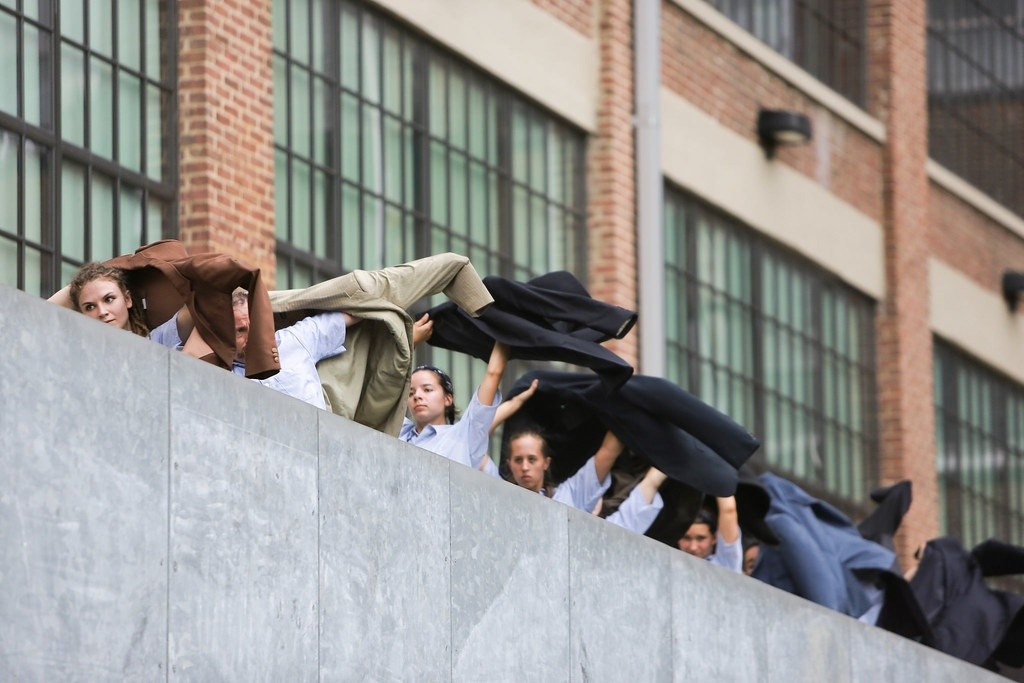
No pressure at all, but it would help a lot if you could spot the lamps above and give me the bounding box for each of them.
[758,109,814,144]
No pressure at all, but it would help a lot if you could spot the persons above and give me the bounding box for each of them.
[196,253,494,439]
[47,240,281,380]
[741,544,759,573]
[489,368,760,512]
[677,487,745,572]
[599,466,668,536]
[399,272,639,470]
